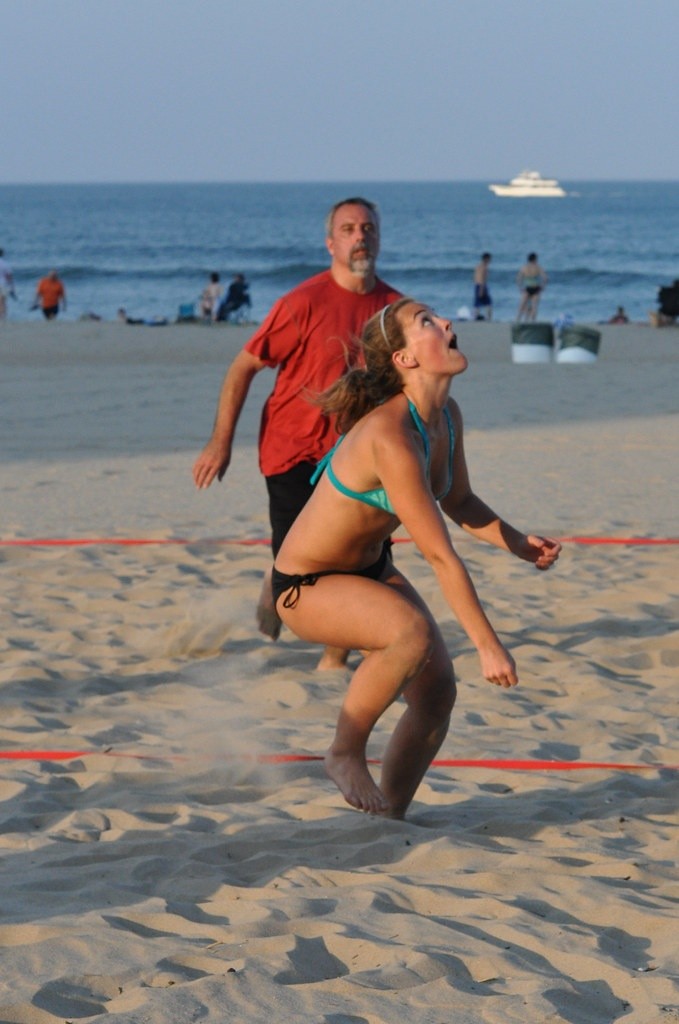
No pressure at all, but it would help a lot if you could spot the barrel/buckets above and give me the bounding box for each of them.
[555,323,602,365]
[511,323,555,363]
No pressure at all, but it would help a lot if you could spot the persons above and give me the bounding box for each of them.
[34,270,67,320]
[216,273,252,322]
[0,249,14,314]
[656,279,679,322]
[118,309,127,319]
[517,254,545,322]
[199,273,223,320]
[195,196,409,670]
[474,253,492,318]
[271,298,563,817]
[608,307,628,323]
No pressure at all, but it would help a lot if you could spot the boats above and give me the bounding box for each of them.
[489,169,566,197]
